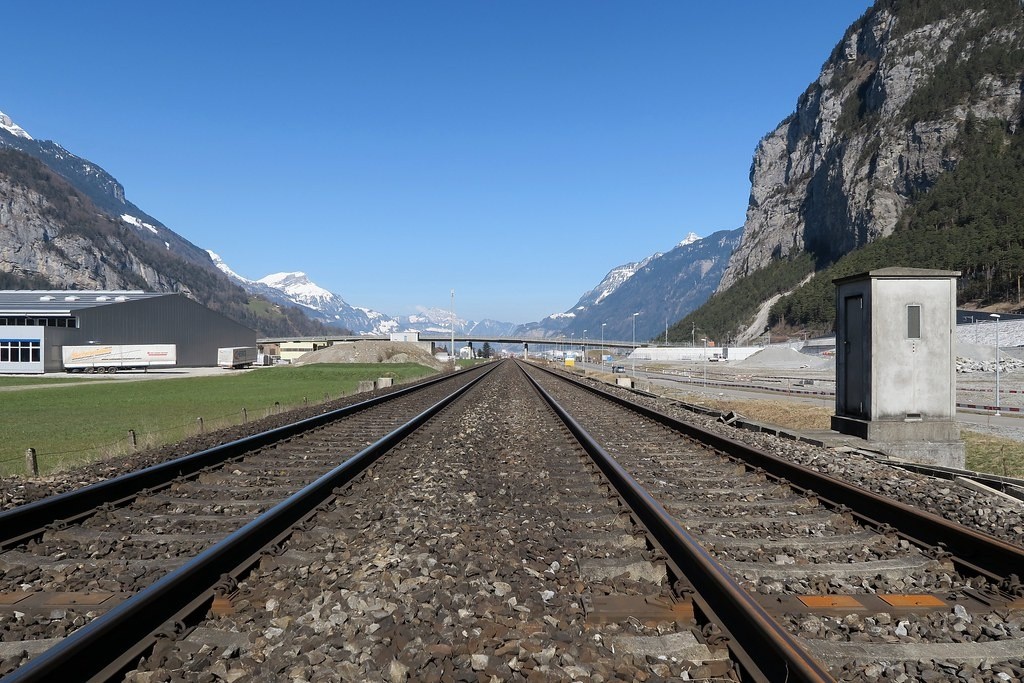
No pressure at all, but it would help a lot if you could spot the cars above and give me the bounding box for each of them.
[616,366,625,373]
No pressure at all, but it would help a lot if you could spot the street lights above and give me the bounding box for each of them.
[601,323,606,373]
[692,321,695,347]
[450,288,456,357]
[555,330,588,365]
[989,314,1001,416]
[632,313,640,376]
[701,339,706,386]
